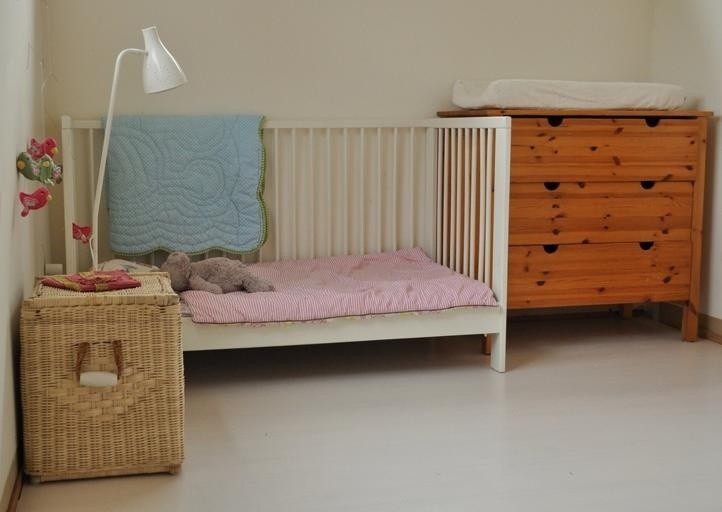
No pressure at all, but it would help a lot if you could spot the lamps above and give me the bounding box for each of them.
[85,22,189,275]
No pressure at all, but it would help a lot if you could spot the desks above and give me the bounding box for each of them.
[19,271,185,485]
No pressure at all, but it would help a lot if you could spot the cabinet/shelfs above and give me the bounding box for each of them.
[436,107,713,354]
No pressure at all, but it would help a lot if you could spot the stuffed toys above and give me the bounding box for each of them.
[17,137,63,217]
[149,252,275,295]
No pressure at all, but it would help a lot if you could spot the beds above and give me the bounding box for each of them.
[61,113,511,376]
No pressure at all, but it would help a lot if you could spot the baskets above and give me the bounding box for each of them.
[20,306,187,486]
